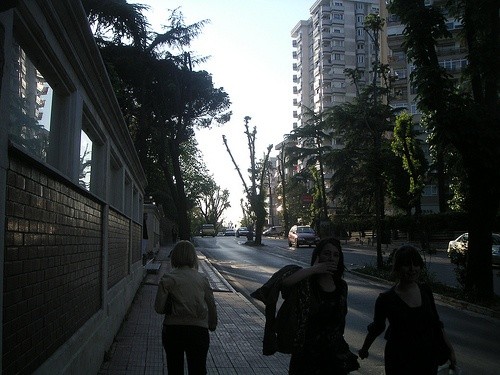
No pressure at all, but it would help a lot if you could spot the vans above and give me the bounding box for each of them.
[262,225,285,237]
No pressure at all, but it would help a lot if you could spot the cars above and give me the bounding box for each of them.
[217,231,225,237]
[236,227,256,238]
[288,224,321,248]
[446,232,500,265]
[224,228,236,236]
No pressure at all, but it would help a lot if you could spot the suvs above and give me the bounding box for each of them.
[200,224,216,238]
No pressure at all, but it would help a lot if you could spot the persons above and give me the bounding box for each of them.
[359,246,457,375]
[281,239,348,375]
[142,212,149,254]
[155,241,218,375]
